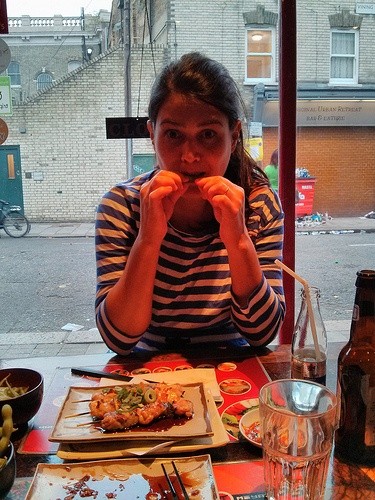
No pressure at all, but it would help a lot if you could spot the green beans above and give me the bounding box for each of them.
[0,403,18,471]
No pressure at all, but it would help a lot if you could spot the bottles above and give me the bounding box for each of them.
[333,270,375,467]
[290,286,328,388]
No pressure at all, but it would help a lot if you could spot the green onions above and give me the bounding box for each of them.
[102,385,156,413]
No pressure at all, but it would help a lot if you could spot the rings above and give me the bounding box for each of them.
[225,187,229,193]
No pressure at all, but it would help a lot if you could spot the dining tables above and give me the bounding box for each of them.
[0,342,375,500]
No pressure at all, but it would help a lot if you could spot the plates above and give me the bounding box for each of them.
[223,398,259,440]
[55,387,231,460]
[45,380,214,441]
[24,454,220,500]
[219,378,252,395]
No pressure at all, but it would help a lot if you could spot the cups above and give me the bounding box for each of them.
[257,379,337,500]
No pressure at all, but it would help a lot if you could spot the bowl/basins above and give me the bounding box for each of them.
[239,408,262,449]
[0,440,17,499]
[0,367,44,427]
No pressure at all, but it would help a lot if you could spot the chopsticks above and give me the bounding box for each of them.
[160,461,190,500]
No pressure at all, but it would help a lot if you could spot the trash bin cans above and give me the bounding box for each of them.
[294,175,316,216]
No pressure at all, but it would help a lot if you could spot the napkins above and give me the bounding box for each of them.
[100,368,222,403]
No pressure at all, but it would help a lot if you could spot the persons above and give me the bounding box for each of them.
[93,52,287,356]
[264,148,279,192]
